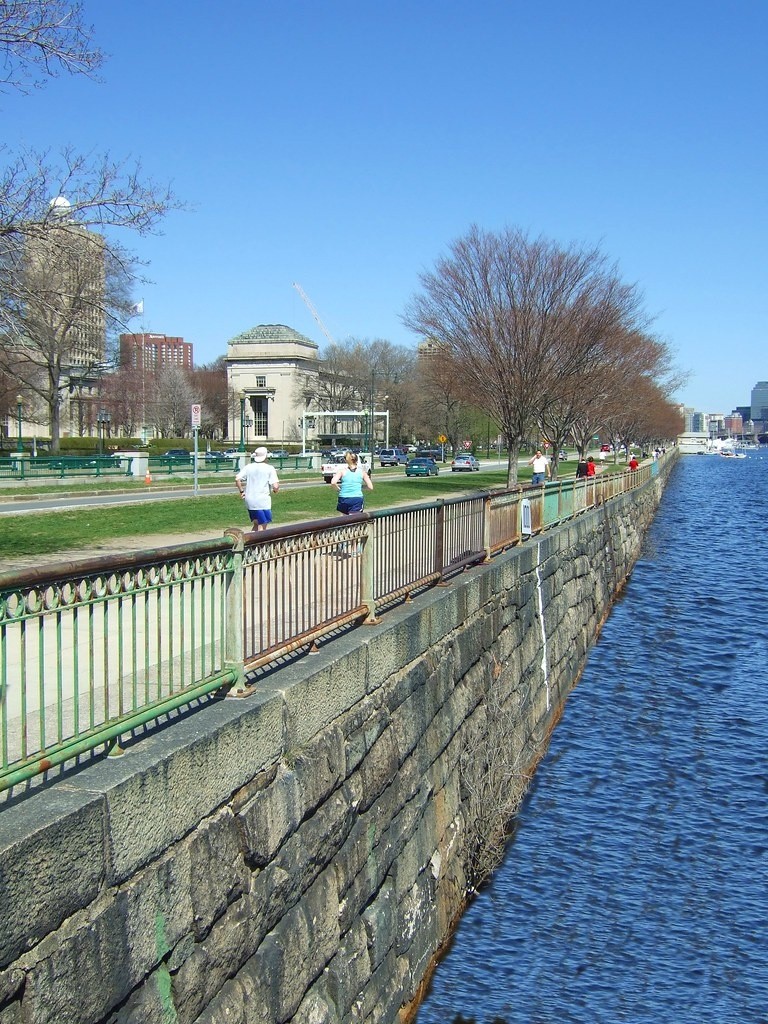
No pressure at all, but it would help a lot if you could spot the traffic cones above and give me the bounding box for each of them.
[144,469,151,484]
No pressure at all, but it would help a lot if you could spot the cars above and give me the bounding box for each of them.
[404,444,417,453]
[404,458,440,477]
[378,449,409,466]
[396,444,409,454]
[600,444,610,453]
[224,448,239,462]
[550,450,567,462]
[451,456,480,471]
[160,450,194,465]
[46,454,119,470]
[251,450,273,458]
[272,450,289,457]
[205,452,224,464]
[319,445,392,459]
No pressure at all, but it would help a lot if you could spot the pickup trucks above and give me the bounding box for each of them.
[415,445,448,463]
[299,449,322,457]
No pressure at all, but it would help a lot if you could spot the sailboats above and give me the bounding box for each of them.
[697,428,747,459]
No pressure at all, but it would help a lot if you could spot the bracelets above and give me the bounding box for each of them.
[240,490,244,493]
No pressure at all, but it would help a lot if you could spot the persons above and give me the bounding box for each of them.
[331,452,374,556]
[587,456,596,476]
[599,449,605,467]
[235,447,279,530]
[655,447,665,457]
[629,457,638,470]
[576,459,587,478]
[529,450,550,484]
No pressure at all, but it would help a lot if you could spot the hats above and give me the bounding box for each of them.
[254,446,267,463]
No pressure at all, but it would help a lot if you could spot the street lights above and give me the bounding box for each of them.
[370,371,398,470]
[16,395,24,453]
[237,391,246,452]
